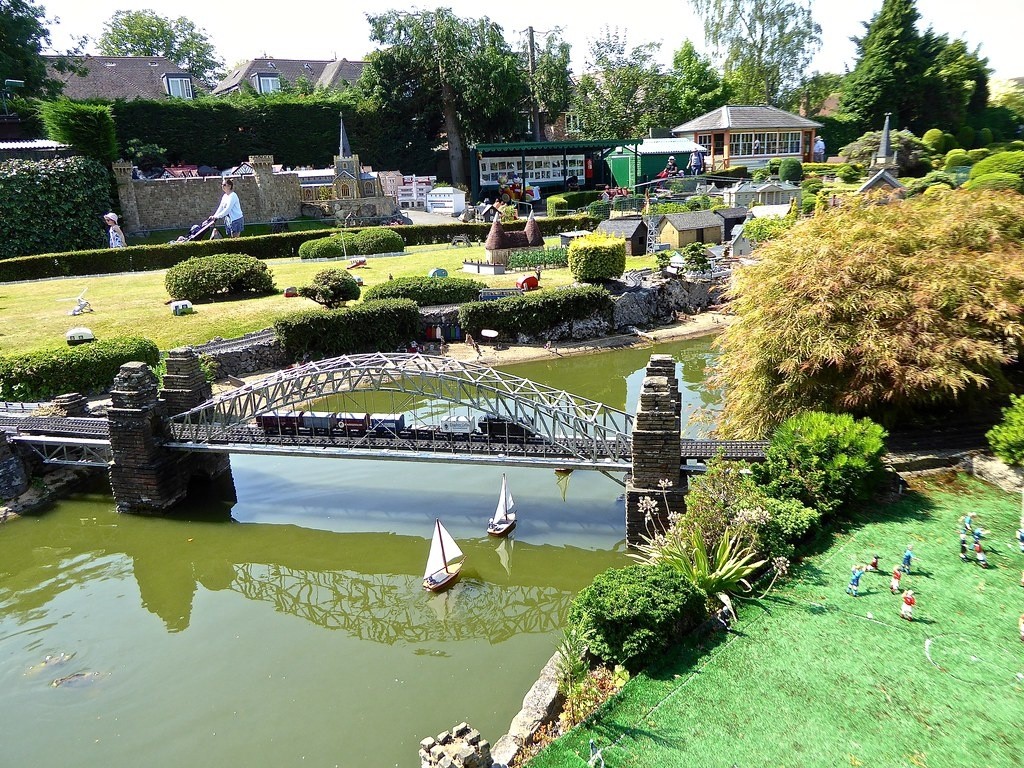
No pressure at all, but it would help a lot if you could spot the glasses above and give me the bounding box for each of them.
[104,218,110,222]
[220,183,226,187]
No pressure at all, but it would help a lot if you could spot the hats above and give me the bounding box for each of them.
[668,156,674,159]
[692,148,698,152]
[104,212,119,225]
[815,136,823,140]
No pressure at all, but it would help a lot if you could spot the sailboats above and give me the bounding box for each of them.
[485,471,518,537]
[421,517,467,592]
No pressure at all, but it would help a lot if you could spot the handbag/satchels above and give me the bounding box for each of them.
[210,228,224,240]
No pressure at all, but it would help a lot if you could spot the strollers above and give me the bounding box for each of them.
[170,217,219,243]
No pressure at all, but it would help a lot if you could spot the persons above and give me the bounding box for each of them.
[104,213,127,248]
[845,544,928,621]
[954,511,990,568]
[655,147,704,178]
[1015,517,1024,554]
[814,136,825,163]
[176,179,244,242]
[566,176,580,192]
